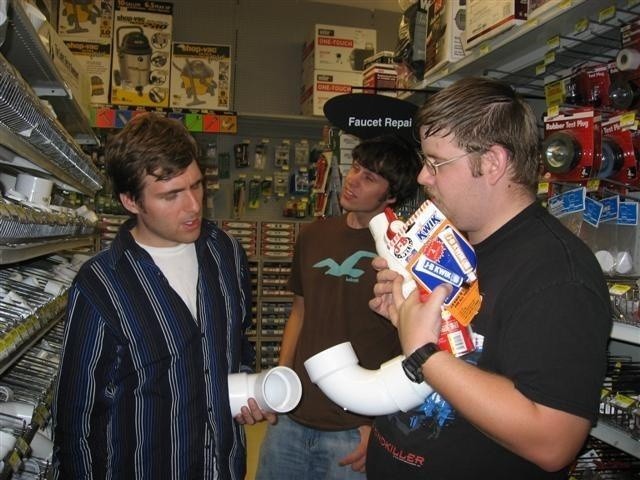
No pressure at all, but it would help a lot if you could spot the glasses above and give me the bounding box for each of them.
[416,148,483,176]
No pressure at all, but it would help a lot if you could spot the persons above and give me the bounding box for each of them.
[364,73,614,480]
[50,114,279,480]
[252,132,420,480]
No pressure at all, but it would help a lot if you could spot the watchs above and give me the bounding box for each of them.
[400,341,444,384]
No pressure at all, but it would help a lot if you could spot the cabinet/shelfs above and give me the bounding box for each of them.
[590,320,640,459]
[95,214,311,371]
[0,0,107,480]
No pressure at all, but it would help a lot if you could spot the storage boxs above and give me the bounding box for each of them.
[58,0,231,110]
[302,0,562,117]
